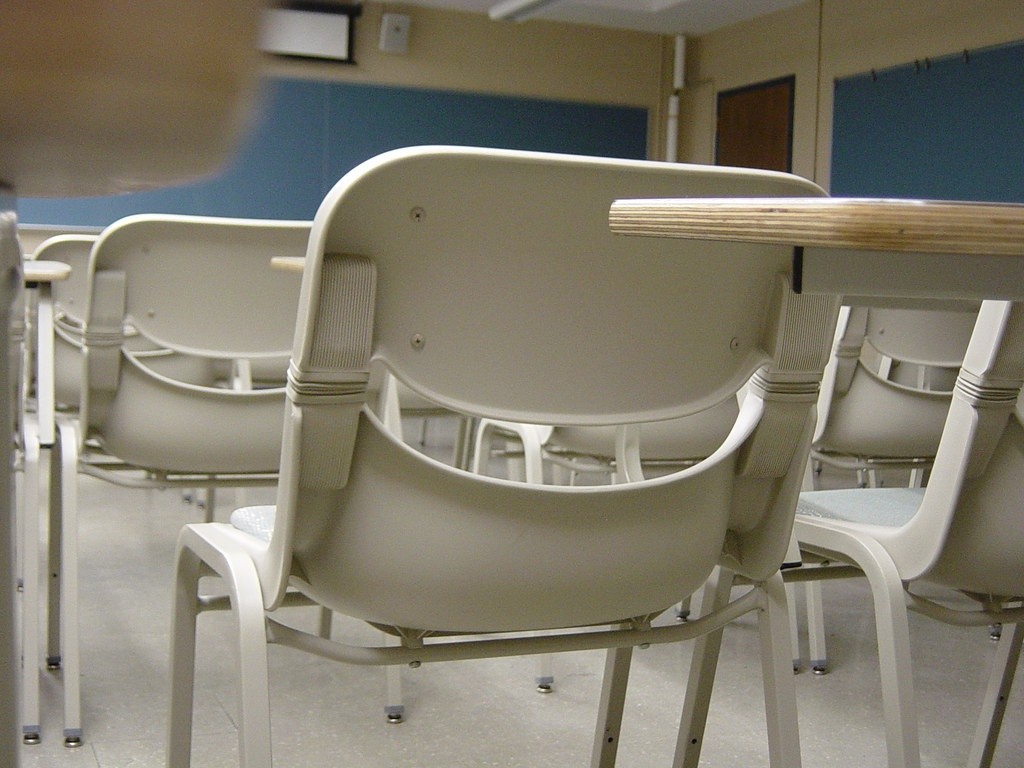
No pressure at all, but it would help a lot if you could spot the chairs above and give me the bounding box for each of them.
[1,145,1023,768]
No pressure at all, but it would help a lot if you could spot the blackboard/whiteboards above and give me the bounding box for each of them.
[1,67,650,235]
[829,38,1024,202]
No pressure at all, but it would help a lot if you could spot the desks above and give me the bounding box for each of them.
[606,198,1023,306]
